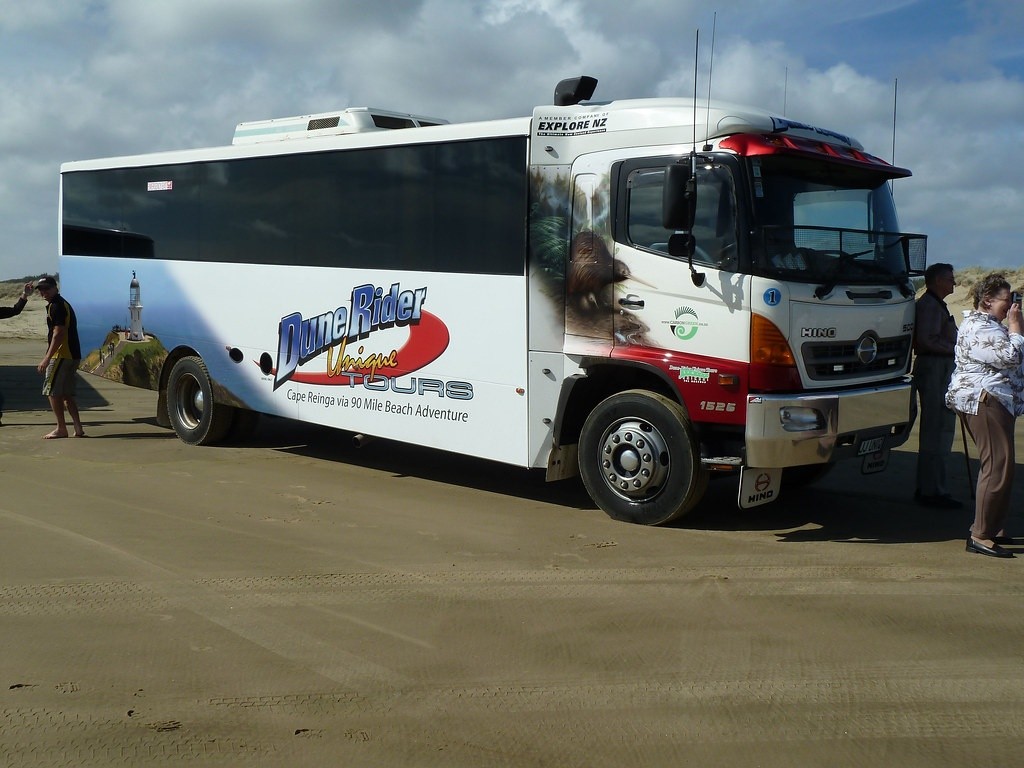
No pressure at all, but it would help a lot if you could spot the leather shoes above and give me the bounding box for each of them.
[966,537,1013,558]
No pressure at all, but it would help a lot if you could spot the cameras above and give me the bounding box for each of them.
[1013,293,1023,310]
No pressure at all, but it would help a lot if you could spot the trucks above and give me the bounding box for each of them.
[57,75,928,527]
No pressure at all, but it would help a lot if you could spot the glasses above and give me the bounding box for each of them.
[944,275,955,280]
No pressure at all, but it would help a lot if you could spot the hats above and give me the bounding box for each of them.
[34,277,57,290]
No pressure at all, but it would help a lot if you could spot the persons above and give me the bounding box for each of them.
[35,275,85,439]
[913,264,957,508]
[945,273,1024,558]
[0,280,35,427]
[99,338,114,366]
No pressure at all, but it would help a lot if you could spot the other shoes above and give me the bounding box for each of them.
[912,489,952,509]
